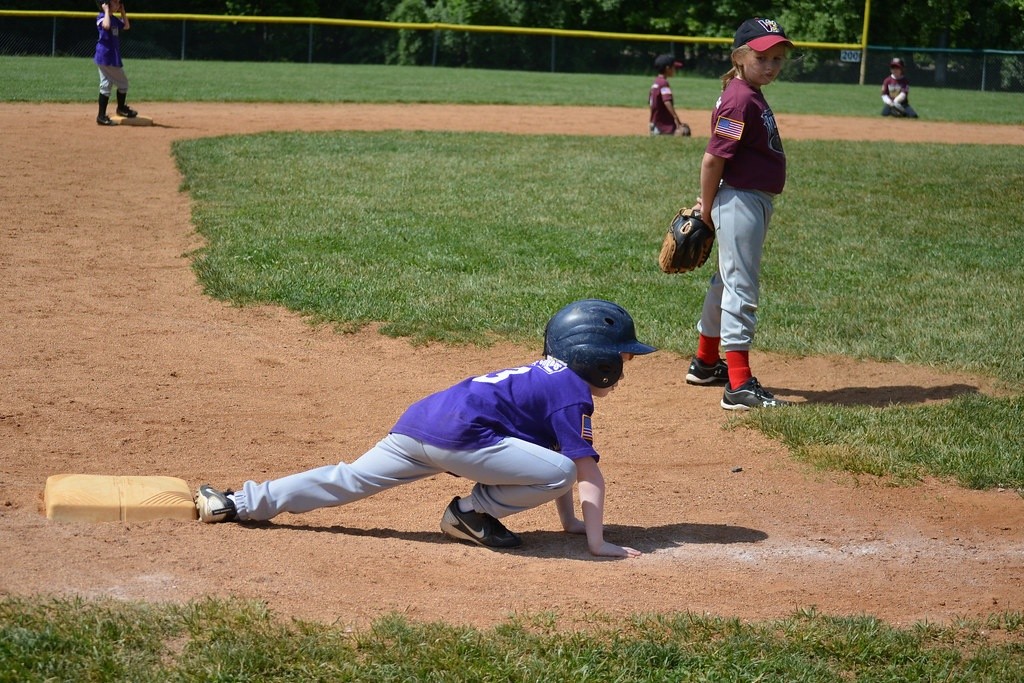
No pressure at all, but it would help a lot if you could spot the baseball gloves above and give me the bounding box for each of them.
[674,123,692,138]
[658,206,714,273]
[891,104,906,117]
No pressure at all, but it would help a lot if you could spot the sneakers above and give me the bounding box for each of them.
[116,105,137,117]
[194,484,234,523]
[440,496,523,549]
[96,115,118,126]
[720,376,797,411]
[685,356,729,386]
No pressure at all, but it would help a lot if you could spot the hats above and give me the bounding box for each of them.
[654,54,683,68]
[733,16,794,52]
[889,58,905,70]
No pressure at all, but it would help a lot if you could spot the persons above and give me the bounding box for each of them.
[197,299,658,558]
[96,0,138,126]
[650,55,691,136]
[686,17,796,413]
[881,58,919,118]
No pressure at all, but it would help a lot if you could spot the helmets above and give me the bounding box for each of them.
[541,299,660,389]
